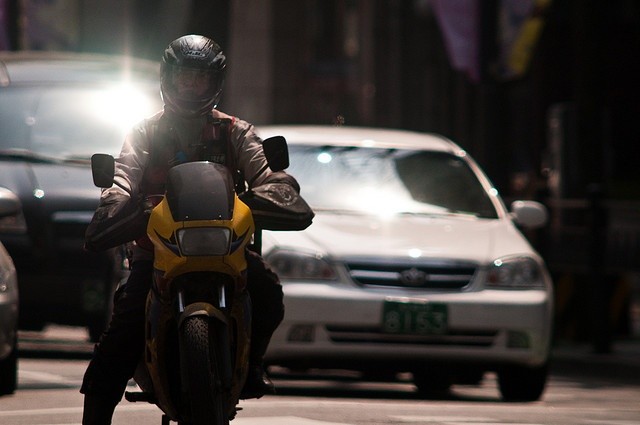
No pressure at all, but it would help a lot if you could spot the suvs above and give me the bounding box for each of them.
[1,50,164,334]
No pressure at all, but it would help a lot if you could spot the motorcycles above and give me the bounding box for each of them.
[91,135,289,425]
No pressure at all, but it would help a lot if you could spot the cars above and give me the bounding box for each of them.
[180,123,553,402]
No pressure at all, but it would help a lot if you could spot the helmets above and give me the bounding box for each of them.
[159,34,227,119]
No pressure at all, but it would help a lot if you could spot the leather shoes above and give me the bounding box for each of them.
[246,352,275,390]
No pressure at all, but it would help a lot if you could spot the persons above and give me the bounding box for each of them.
[79,34,302,425]
